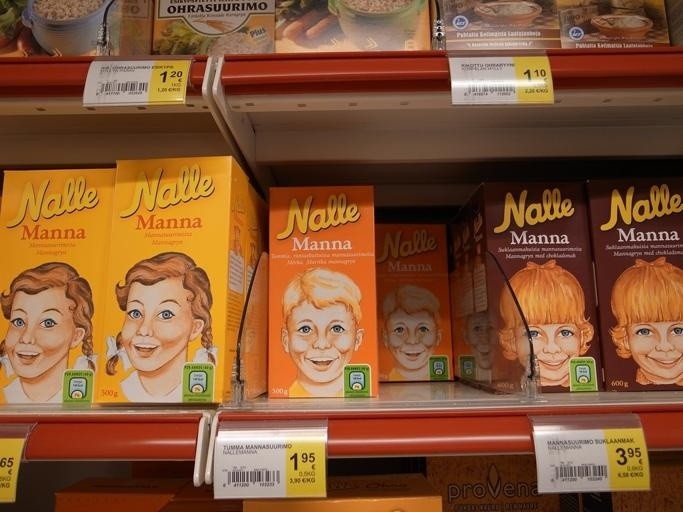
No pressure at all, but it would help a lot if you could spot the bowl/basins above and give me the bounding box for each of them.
[472,1,544,26]
[20,1,119,56]
[590,14,655,40]
[327,0,427,42]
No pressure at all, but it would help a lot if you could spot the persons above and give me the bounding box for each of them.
[461,289,499,389]
[606,257,682,389]
[278,267,365,398]
[103,253,218,402]
[0,262,98,403]
[378,285,443,382]
[497,259,593,389]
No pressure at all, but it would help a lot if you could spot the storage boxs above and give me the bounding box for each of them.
[2,2,681,59]
[2,154,680,406]
[56,453,679,508]
